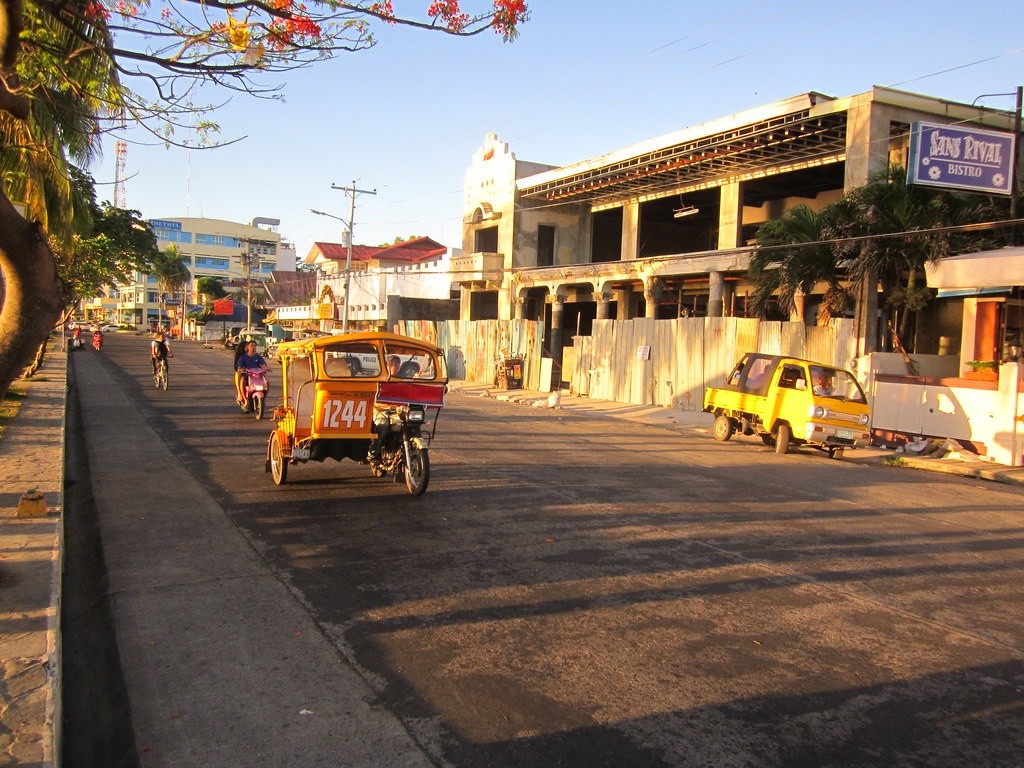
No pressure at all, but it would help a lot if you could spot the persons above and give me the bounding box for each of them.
[72,323,82,340]
[234,341,271,409]
[93,324,104,347]
[813,372,839,396]
[735,363,770,383]
[312,332,317,338]
[152,332,172,375]
[368,355,410,465]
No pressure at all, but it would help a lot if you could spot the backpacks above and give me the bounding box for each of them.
[155,340,167,358]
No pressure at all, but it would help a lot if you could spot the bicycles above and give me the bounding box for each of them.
[151,352,174,391]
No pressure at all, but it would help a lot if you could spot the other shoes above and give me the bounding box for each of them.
[237,397,243,401]
[242,404,248,410]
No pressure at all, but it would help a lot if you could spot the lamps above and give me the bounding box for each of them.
[672,205,699,218]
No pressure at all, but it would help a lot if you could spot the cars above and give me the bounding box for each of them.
[67,322,90,333]
[100,325,120,333]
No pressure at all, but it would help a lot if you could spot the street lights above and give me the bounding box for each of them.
[312,209,354,330]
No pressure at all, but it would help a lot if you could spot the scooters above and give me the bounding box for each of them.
[92,328,103,353]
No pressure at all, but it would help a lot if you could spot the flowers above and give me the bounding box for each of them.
[966,360,999,372]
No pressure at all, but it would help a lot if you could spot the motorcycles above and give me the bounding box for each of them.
[234,367,272,420]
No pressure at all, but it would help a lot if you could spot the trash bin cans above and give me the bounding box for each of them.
[505,359,525,390]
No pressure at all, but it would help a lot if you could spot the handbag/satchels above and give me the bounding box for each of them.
[74,339,80,347]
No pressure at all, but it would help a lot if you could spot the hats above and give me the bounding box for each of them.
[387,356,400,363]
[401,362,419,373]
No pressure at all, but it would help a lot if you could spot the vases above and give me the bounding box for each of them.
[964,372,998,382]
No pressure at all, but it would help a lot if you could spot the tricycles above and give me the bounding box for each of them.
[265,331,450,496]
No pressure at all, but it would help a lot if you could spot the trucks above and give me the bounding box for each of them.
[700,352,871,460]
